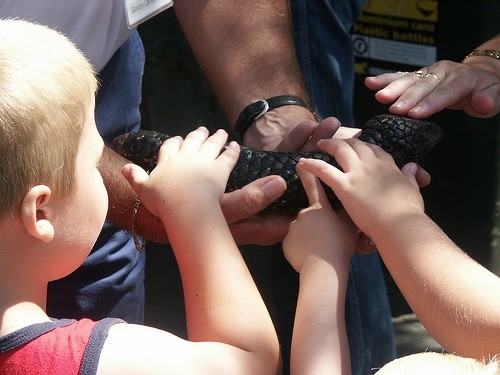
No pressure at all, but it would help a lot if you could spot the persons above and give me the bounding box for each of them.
[363,36,500,117]
[351,0,500,271]
[280,163,500,375]
[0,0,432,326]
[289,0,396,375]
[0,19,283,375]
[298,138,500,366]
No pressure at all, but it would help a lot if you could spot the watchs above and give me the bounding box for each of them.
[231,95,321,144]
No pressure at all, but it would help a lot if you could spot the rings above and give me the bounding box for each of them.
[413,71,423,78]
[397,71,409,76]
[424,73,441,84]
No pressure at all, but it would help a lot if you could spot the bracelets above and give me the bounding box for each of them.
[465,49,500,61]
[130,196,147,252]
